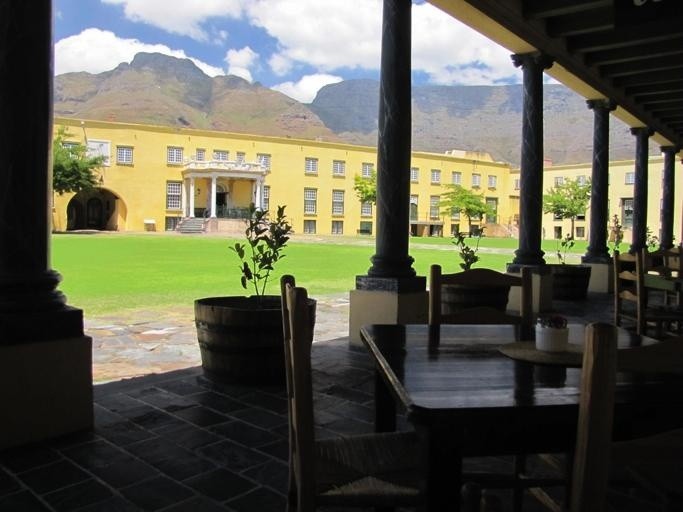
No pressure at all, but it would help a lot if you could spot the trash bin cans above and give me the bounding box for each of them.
[147,224,156,231]
[470,229,481,236]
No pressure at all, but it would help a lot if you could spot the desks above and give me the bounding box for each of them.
[356,319,681,510]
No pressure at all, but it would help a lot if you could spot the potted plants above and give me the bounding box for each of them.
[440,225,509,314]
[544,233,591,302]
[533,313,571,353]
[193,201,315,387]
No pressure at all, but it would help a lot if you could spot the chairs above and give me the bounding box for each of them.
[280,271,482,510]
[511,322,681,512]
[609,248,681,340]
